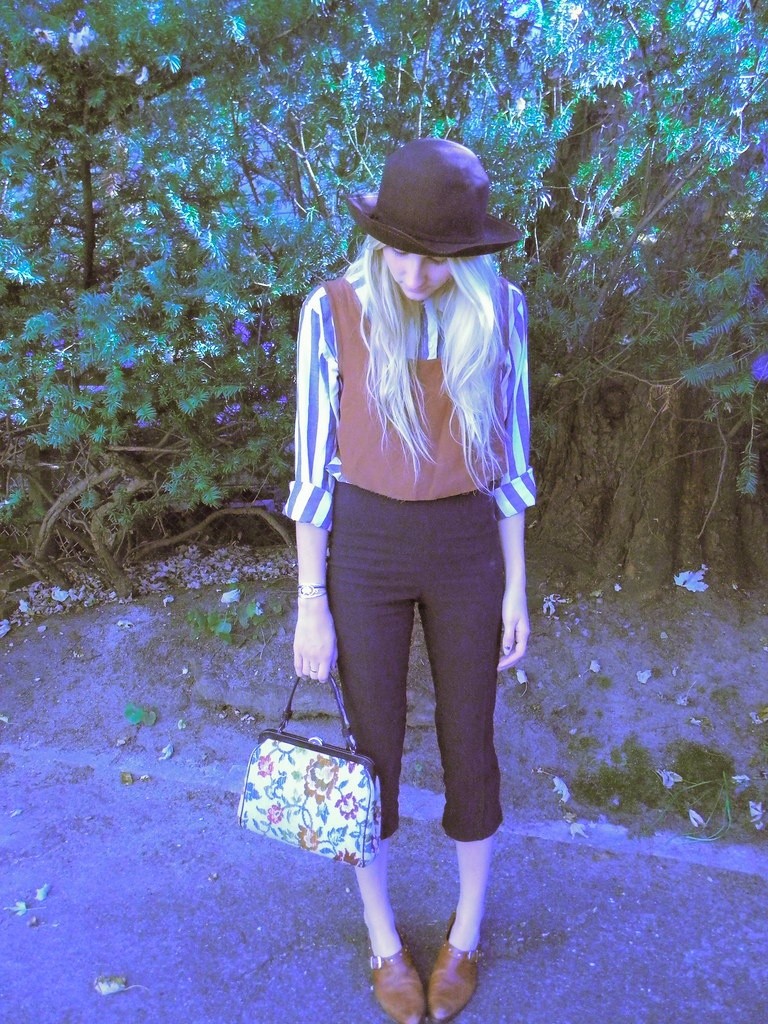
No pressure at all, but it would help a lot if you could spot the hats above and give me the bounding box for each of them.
[344,138,523,257]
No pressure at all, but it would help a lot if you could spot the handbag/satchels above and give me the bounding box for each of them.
[237,671,382,868]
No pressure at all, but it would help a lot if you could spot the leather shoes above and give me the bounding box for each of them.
[427,912,484,1024]
[368,920,427,1024]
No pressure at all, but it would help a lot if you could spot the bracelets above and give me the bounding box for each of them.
[297,584,327,599]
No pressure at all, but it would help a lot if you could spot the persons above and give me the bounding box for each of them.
[282,138,537,1024]
